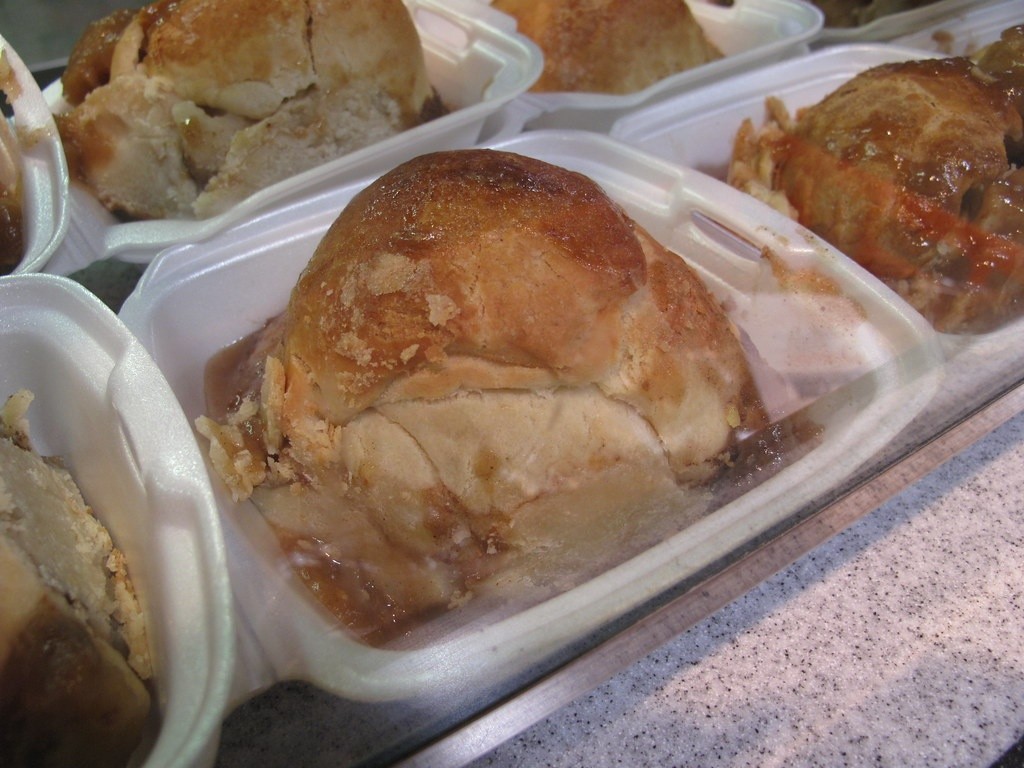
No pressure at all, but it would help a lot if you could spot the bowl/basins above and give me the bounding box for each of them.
[0,2,1024,768]
